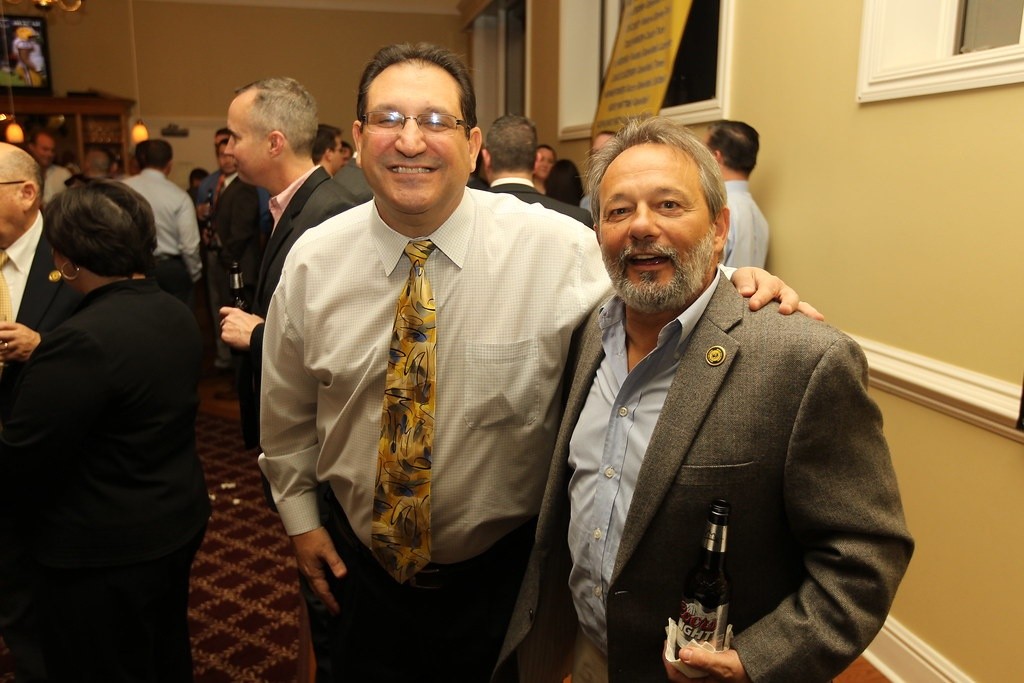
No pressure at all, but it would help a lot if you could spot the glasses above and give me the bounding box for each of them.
[363,111,470,134]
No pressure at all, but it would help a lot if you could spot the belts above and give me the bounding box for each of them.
[326,490,526,589]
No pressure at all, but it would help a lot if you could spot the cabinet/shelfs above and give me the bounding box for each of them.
[0,93,137,177]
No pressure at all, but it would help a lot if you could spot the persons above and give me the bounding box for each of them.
[0,178,212,683]
[258,45,824,683]
[0,140,86,432]
[29,75,769,515]
[493,114,915,683]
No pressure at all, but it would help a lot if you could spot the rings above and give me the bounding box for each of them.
[4,342,9,350]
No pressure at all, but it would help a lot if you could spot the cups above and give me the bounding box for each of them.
[0,314,7,344]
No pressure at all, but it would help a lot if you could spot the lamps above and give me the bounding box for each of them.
[0,114,24,144]
[131,119,149,145]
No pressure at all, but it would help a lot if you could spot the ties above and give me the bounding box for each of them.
[217,182,224,198]
[371,240,437,583]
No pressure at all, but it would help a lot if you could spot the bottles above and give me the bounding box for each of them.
[226,268,248,316]
[207,220,214,240]
[675,494,735,657]
[203,188,213,217]
